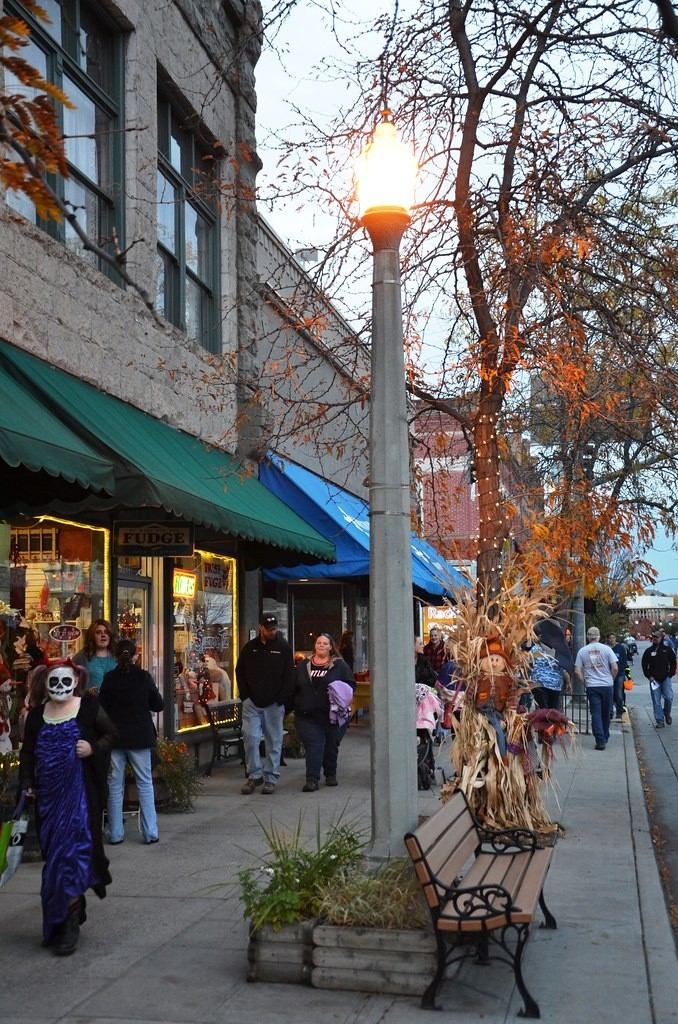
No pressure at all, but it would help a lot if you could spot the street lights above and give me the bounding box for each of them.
[357,107,428,862]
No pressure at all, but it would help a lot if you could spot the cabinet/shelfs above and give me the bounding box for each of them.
[26,617,87,661]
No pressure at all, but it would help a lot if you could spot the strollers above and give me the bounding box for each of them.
[413,683,439,790]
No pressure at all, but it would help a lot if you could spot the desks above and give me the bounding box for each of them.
[347,681,370,728]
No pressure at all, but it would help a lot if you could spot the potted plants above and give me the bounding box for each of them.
[304,836,441,997]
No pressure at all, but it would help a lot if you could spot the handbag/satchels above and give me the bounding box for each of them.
[1,791,35,887]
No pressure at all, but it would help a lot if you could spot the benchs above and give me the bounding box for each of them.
[404,788,557,1018]
[201,698,287,778]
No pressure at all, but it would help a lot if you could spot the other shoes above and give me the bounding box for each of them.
[665,714,673,725]
[656,722,666,728]
[241,777,264,795]
[261,783,276,794]
[43,921,82,955]
[594,744,606,750]
[302,778,319,791]
[325,776,338,786]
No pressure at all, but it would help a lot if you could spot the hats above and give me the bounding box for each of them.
[651,631,663,638]
[587,627,600,639]
[259,613,279,630]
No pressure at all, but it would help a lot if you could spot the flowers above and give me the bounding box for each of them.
[180,793,380,940]
[0,600,28,657]
[126,734,204,813]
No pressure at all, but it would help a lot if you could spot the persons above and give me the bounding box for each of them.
[670,633,678,653]
[528,645,572,743]
[339,630,355,672]
[236,613,294,795]
[415,636,441,780]
[208,625,233,678]
[439,661,467,783]
[623,665,631,712]
[72,620,117,695]
[575,627,618,749]
[605,632,628,722]
[294,634,356,792]
[424,628,449,674]
[98,640,165,844]
[462,634,517,766]
[15,658,117,956]
[642,631,676,728]
[660,628,675,653]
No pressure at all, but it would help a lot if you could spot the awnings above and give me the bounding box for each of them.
[0,342,336,571]
[259,453,476,607]
[0,364,114,517]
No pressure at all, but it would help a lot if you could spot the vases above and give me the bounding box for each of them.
[126,769,173,812]
[248,917,322,982]
